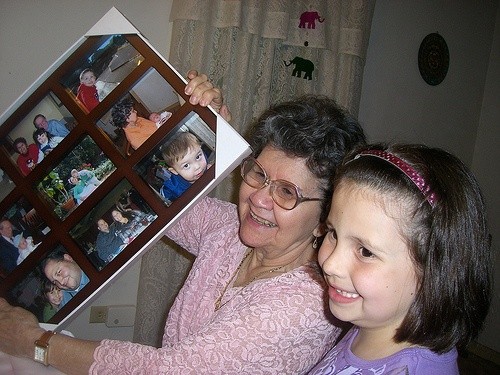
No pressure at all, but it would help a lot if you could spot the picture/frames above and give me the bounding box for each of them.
[49,91,63,108]
[0,6,253,336]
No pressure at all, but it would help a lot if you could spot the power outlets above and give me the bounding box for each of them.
[89,304,108,324]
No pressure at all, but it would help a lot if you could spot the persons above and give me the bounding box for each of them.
[149,111,173,128]
[160,131,212,202]
[0,69,368,375]
[41,252,91,322]
[68,169,100,204]
[76,69,101,112]
[95,208,131,261]
[306,141,490,375]
[13,114,70,177]
[111,98,159,150]
[0,220,42,274]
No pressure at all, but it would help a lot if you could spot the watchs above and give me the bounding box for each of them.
[32,330,57,367]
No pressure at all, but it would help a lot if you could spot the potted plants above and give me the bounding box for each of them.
[37,171,77,214]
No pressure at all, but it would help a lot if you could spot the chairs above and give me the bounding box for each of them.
[24,208,48,232]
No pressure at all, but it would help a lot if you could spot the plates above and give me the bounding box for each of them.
[418,32,450,86]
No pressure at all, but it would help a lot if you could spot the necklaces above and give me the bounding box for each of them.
[214,247,288,311]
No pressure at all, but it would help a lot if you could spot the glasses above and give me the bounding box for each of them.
[240,156,323,210]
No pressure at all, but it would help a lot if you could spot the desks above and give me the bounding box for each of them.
[119,214,158,253]
